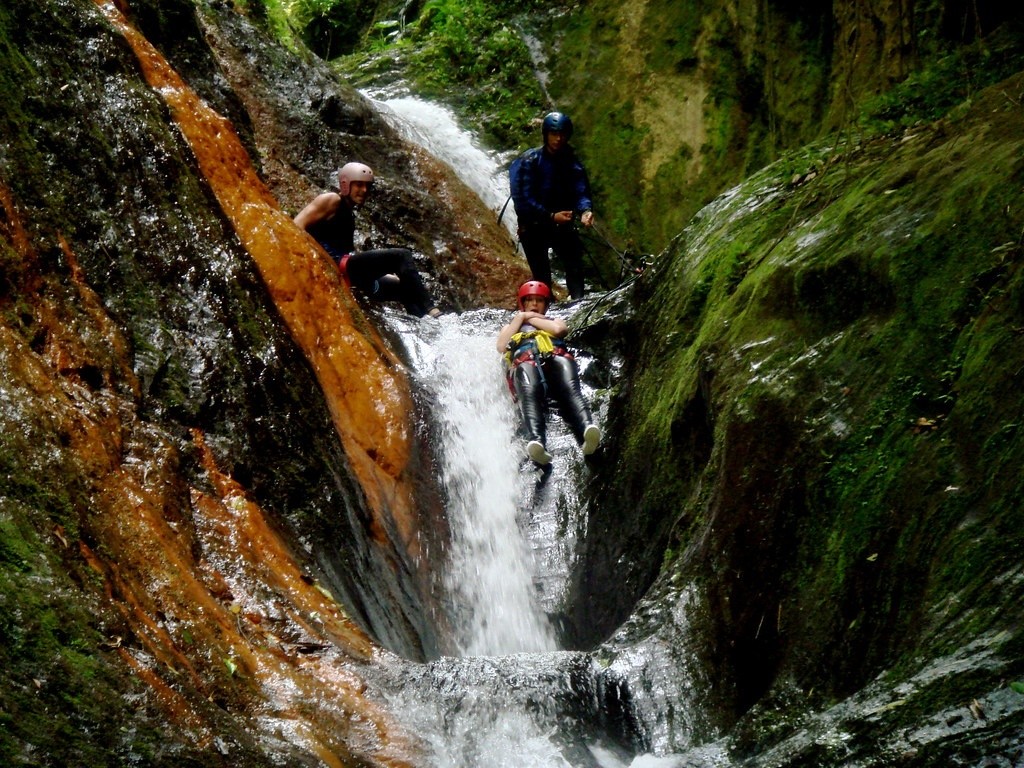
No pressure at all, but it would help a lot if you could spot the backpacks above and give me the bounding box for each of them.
[497,144,578,228]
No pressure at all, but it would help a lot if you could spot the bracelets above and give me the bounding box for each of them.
[551,212,555,220]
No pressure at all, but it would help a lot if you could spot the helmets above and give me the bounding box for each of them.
[542,111,574,134]
[338,162,375,197]
[517,281,550,312]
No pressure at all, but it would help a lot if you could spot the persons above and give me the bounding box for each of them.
[293,162,443,319]
[496,280,601,465]
[509,111,595,304]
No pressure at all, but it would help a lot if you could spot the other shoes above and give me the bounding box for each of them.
[581,424,600,455]
[426,306,445,319]
[526,440,553,465]
